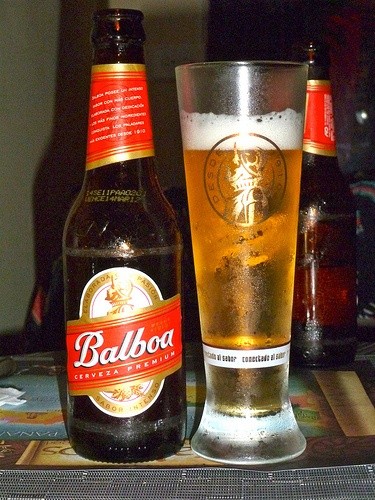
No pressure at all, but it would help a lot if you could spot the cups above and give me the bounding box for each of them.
[174,61,307,463]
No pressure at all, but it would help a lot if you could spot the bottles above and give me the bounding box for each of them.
[289,39,357,370]
[63,8,183,463]
